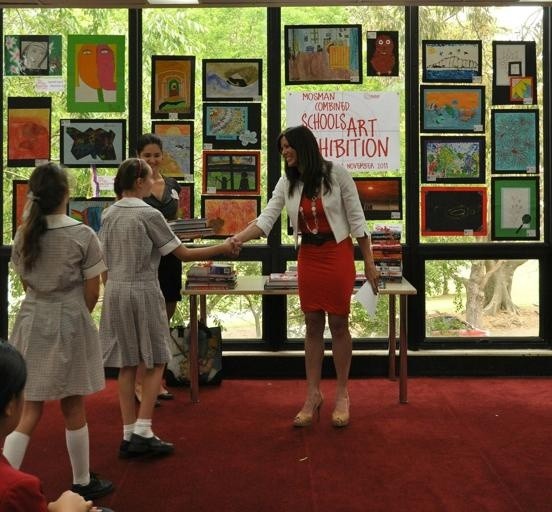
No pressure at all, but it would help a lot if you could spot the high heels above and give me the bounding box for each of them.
[331,392,351,427]
[292,390,324,428]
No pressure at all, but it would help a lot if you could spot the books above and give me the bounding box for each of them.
[170,216,238,291]
[262,225,403,290]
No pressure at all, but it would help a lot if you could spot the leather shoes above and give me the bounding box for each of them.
[69,470,116,502]
[118,431,177,463]
[133,382,175,409]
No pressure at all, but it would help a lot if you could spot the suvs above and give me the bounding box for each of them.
[423,310,492,337]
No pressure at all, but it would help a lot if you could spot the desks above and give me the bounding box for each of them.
[181,276,418,403]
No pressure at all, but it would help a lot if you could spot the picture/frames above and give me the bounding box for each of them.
[60,119,127,170]
[202,58,262,101]
[12,180,31,239]
[367,32,400,77]
[6,97,52,168]
[151,54,195,119]
[489,177,541,240]
[491,109,539,174]
[284,26,363,86]
[4,35,63,77]
[419,135,485,184]
[203,104,262,149]
[422,39,482,82]
[200,197,262,239]
[66,35,126,112]
[420,186,487,238]
[66,195,120,240]
[203,153,261,195]
[492,40,538,105]
[176,181,195,219]
[419,84,486,133]
[151,122,195,175]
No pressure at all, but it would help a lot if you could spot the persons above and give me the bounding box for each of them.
[229,125,380,428]
[2,161,117,499]
[133,133,182,406]
[96,156,242,462]
[0,340,107,512]
